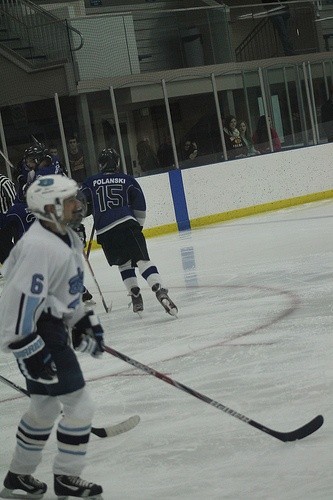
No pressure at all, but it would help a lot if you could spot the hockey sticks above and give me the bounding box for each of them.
[98,342,324,443]
[86,224,96,258]
[0,375,139,439]
[79,247,114,313]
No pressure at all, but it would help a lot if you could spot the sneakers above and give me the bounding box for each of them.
[53,474,104,500]
[130,286,144,317]
[2,471,48,500]
[82,286,97,304]
[156,288,180,318]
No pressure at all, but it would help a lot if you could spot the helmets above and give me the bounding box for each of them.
[98,148,120,172]
[27,174,89,236]
[23,142,44,163]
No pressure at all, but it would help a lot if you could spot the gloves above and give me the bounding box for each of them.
[8,333,61,385]
[72,311,105,358]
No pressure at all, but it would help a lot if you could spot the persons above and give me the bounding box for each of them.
[320,93,333,143]
[0,173,105,500]
[0,115,281,319]
